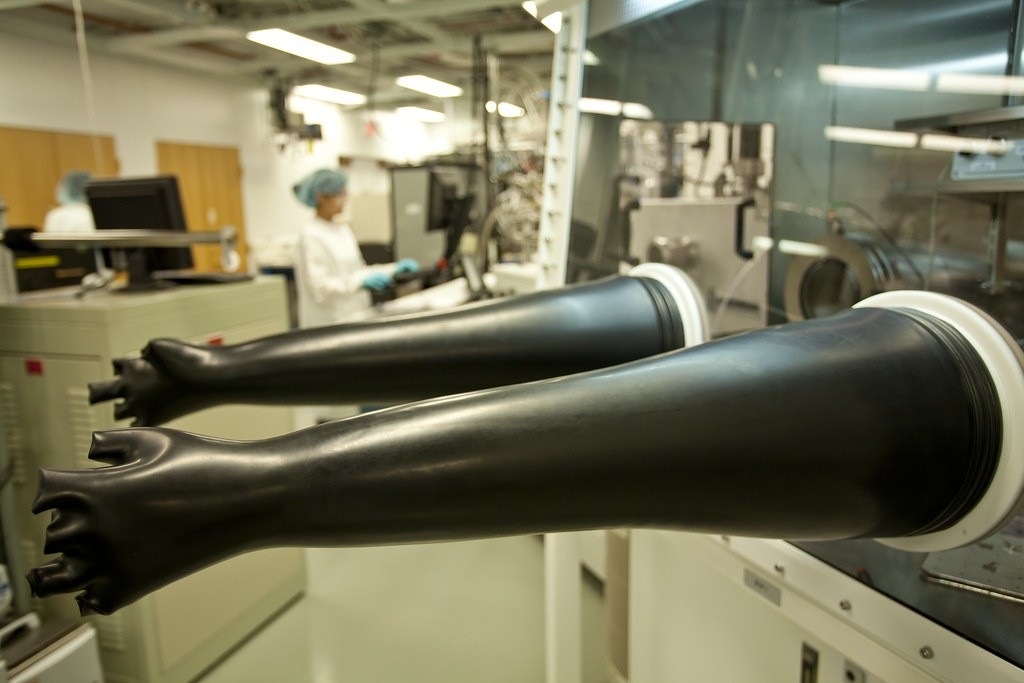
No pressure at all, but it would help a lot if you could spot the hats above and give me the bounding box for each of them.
[294,168,347,205]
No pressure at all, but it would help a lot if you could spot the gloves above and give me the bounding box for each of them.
[366,271,393,293]
[395,257,418,275]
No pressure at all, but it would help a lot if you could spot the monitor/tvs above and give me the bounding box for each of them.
[422,167,485,231]
[84,173,197,274]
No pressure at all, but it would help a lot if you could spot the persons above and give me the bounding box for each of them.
[43,168,97,240]
[293,169,417,335]
